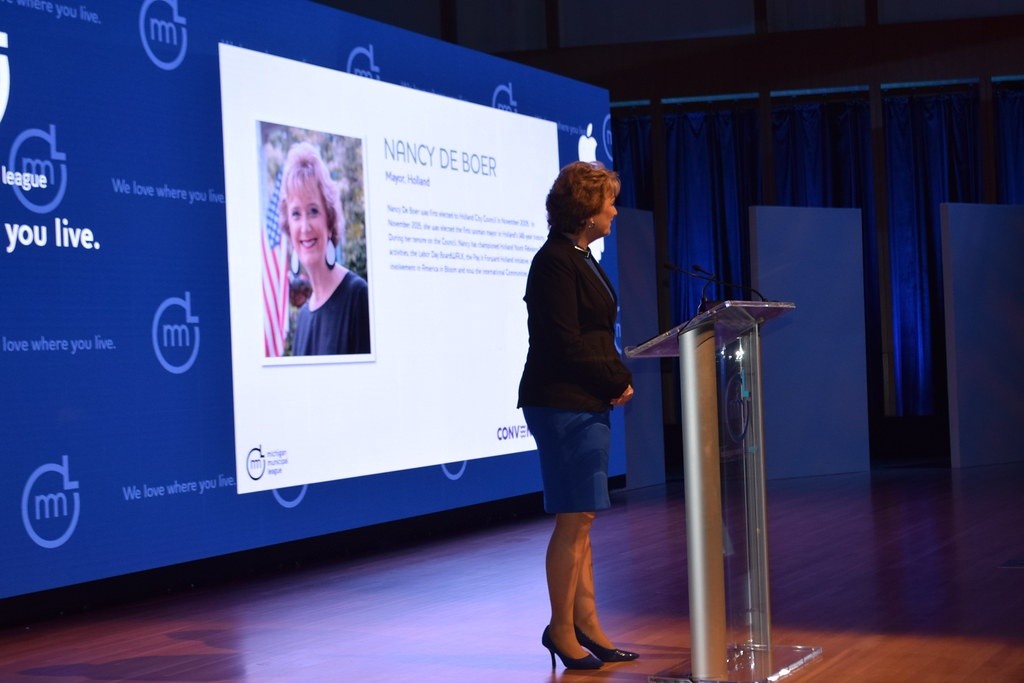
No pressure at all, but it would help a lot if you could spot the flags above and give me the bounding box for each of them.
[262,166,288,356]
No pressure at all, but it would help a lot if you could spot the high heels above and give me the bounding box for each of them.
[541,625,604,670]
[574,622,639,663]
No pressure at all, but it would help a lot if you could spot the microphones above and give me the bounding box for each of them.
[691,266,716,302]
[653,259,763,298]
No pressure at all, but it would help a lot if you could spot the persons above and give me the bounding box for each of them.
[278,142,371,355]
[517,161,640,667]
